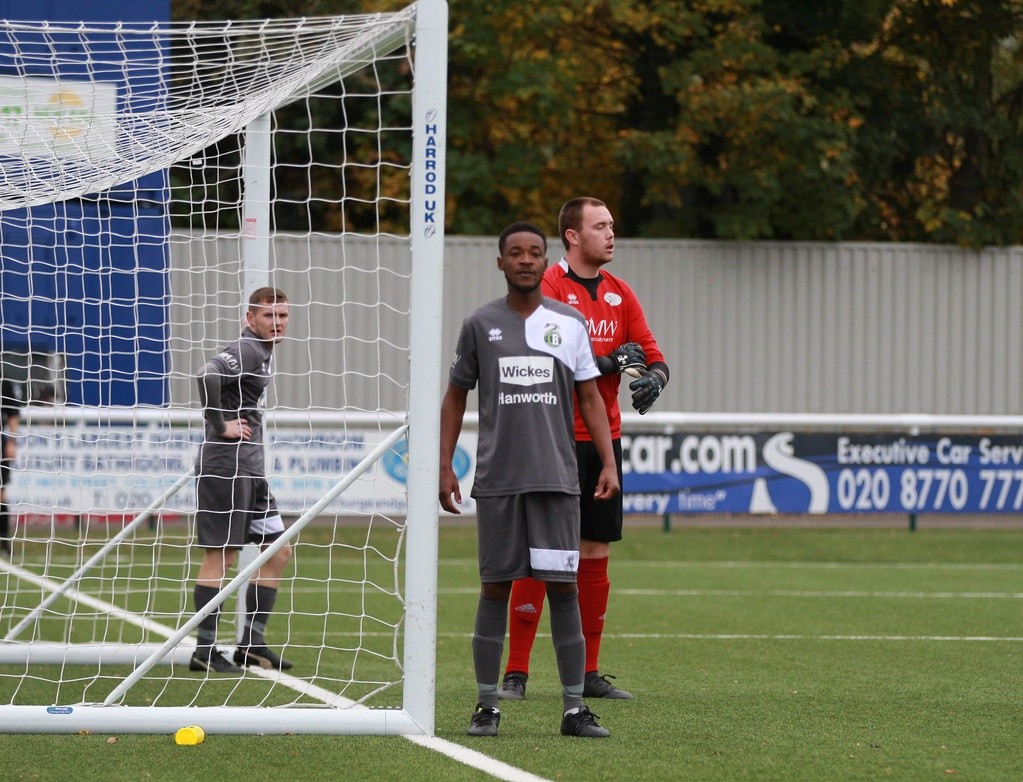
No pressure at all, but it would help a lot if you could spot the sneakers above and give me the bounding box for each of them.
[188,650,249,673]
[559,705,611,738]
[467,701,501,736]
[581,671,633,700]
[498,672,527,700]
[233,643,294,671]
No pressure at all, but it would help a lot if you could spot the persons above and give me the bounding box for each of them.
[189,287,292,673]
[439,222,621,737]
[498,197,670,700]
[0,368,20,557]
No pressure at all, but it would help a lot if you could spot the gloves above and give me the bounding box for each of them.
[628,360,670,416]
[595,342,647,375]
